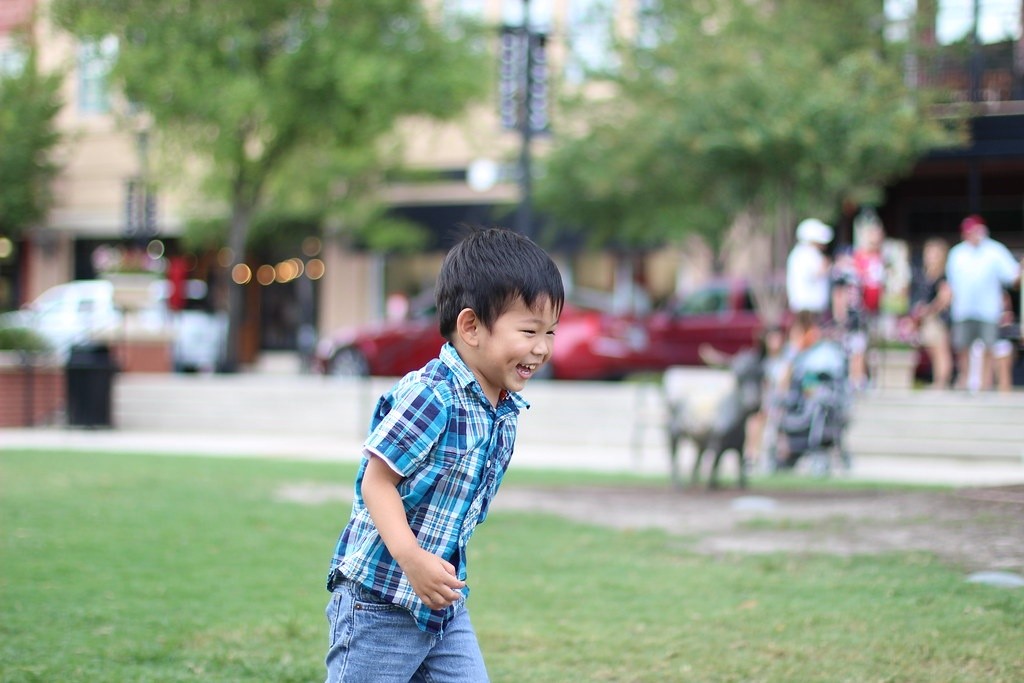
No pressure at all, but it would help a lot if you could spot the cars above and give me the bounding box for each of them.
[631,273,934,382]
[313,283,652,381]
[3,279,215,373]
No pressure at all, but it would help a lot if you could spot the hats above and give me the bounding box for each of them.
[795,218,834,244]
[960,214,985,237]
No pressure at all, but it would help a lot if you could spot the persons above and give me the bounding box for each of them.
[706,203,1023,472]
[312,229,569,681]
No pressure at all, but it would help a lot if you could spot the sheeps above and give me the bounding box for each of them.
[659,346,764,491]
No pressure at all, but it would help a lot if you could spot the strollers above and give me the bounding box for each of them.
[760,338,854,487]
